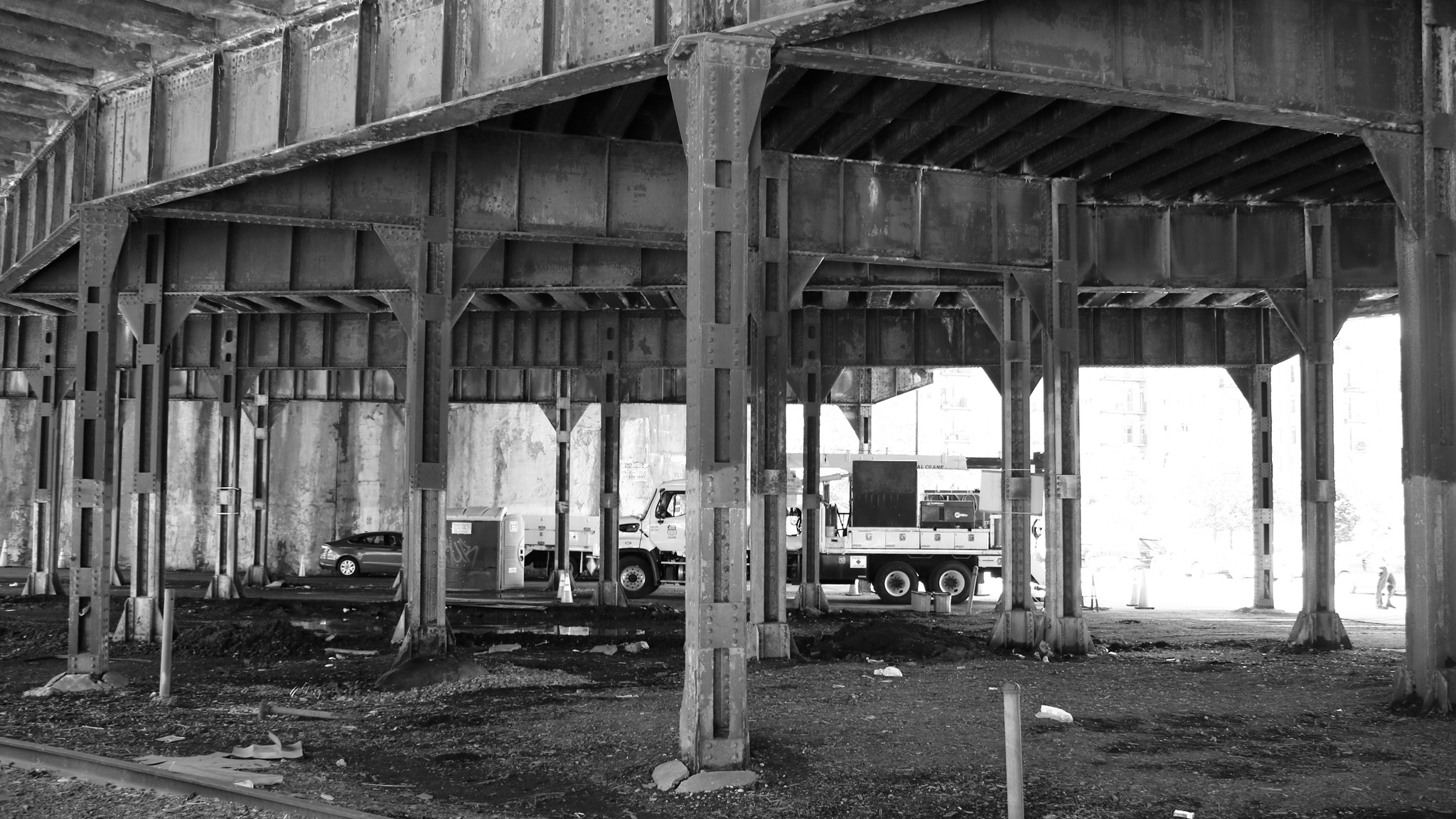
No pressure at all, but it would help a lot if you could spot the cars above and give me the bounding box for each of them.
[1080,537,1303,583]
[318,530,404,578]
[1334,543,1405,595]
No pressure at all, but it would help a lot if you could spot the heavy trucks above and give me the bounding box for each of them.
[618,480,1002,605]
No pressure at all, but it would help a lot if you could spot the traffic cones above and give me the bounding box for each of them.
[0,539,13,568]
[1126,579,1139,607]
[58,547,70,569]
[1134,575,1155,610]
[975,572,990,596]
[845,579,862,596]
[298,555,306,577]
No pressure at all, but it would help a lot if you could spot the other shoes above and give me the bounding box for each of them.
[1386,599,1396,609]
[1375,604,1388,610]
